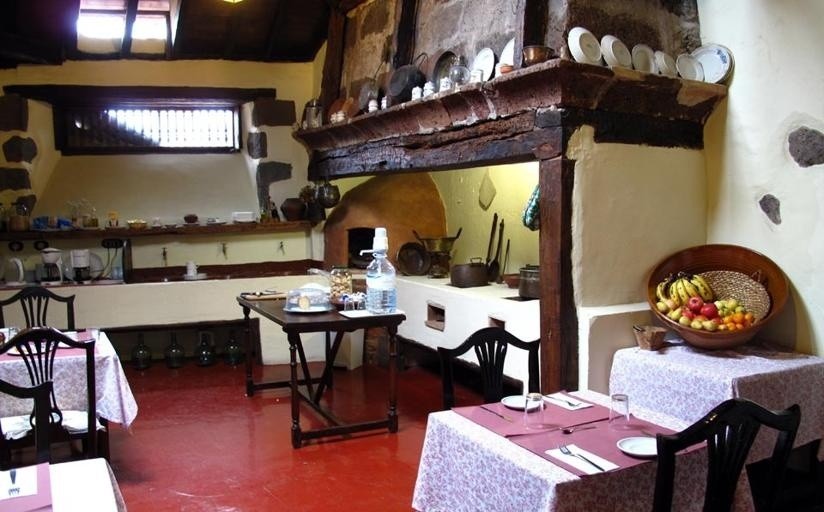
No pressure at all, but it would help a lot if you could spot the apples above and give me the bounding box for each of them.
[683,298,723,324]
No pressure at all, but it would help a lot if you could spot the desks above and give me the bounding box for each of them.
[608,339,824,466]
[236,295,409,448]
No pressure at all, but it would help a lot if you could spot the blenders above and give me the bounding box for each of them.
[70,249,91,284]
[39,248,63,286]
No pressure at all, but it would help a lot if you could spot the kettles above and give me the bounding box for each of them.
[4,258,27,286]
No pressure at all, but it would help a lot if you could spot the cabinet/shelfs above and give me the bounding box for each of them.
[0,221,327,363]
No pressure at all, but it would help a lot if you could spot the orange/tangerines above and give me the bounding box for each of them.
[722,313,754,332]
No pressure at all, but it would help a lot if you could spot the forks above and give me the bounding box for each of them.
[559,445,605,472]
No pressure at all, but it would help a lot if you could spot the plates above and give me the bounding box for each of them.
[501,395,539,411]
[616,436,658,458]
[7,342,72,356]
[430,37,515,93]
[164,221,226,228]
[567,26,735,84]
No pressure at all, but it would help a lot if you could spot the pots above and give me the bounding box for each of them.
[358,52,427,113]
[395,227,540,299]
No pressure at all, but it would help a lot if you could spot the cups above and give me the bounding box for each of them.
[185,261,199,277]
[609,394,630,432]
[524,393,544,431]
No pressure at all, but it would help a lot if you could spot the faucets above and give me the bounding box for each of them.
[161,246,166,258]
[222,243,229,254]
[280,240,286,251]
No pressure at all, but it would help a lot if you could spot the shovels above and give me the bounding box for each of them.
[488,220,504,282]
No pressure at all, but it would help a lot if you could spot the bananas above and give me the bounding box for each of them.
[657,268,713,305]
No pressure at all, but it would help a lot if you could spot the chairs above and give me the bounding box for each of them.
[653,398,801,512]
[437,327,543,409]
[1,287,75,329]
[0,327,96,468]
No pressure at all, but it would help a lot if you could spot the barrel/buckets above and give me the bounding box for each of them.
[365,253,397,313]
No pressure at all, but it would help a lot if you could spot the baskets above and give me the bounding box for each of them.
[667,269,771,327]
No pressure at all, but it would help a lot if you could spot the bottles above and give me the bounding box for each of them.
[329,253,396,315]
[8,327,19,341]
[131,330,240,370]
[0,202,29,230]
[86,326,100,343]
